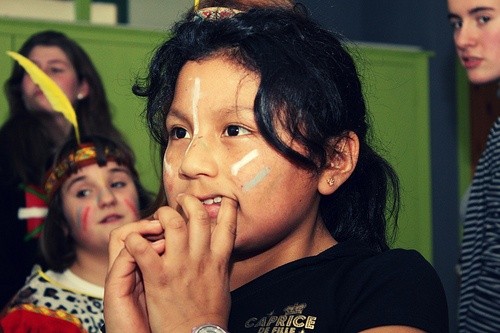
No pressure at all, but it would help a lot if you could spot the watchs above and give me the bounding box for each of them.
[191,321,228,333]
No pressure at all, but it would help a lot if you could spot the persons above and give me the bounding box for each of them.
[0,135,151,332]
[103,3,447,333]
[442,0,500,333]
[0,29,122,313]
